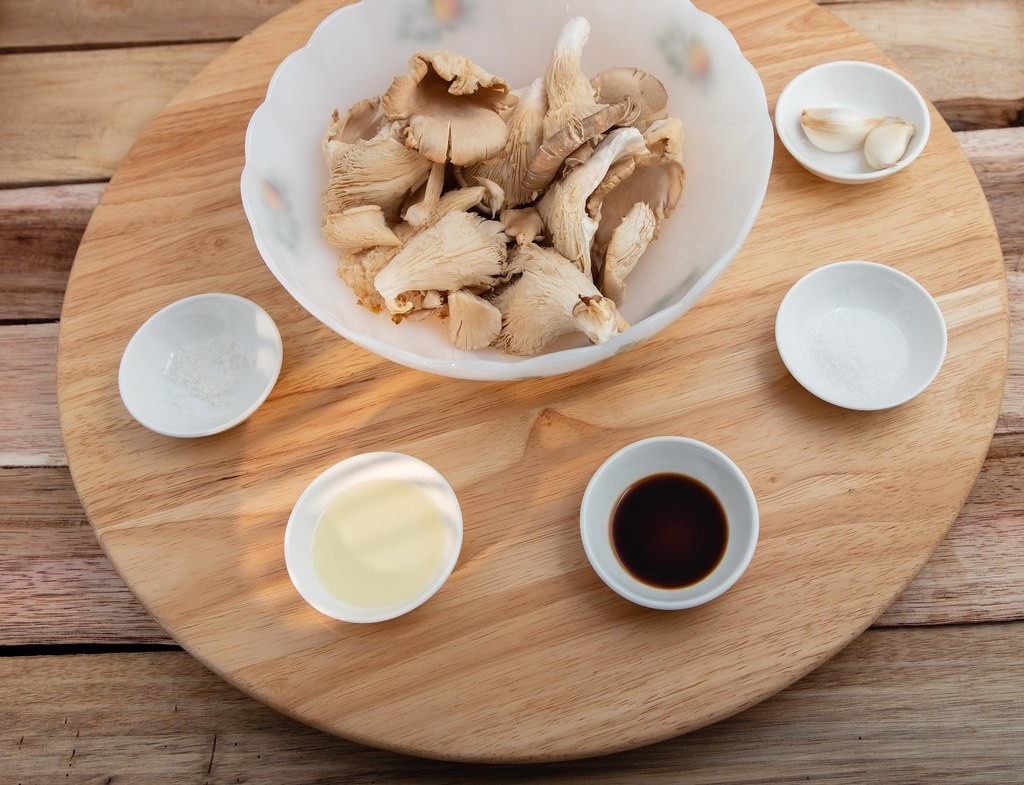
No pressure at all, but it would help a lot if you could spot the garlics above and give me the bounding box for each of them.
[801,108,915,170]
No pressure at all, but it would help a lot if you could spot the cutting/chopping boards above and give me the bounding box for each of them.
[54,0,1016,771]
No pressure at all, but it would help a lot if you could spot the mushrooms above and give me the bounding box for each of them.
[322,17,683,356]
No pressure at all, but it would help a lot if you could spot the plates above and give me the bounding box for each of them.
[775,60,931,185]
[118,294,284,440]
[581,436,759,610]
[282,450,464,624]
[776,261,948,411]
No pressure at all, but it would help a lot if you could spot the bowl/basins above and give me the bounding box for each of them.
[239,0,775,382]
